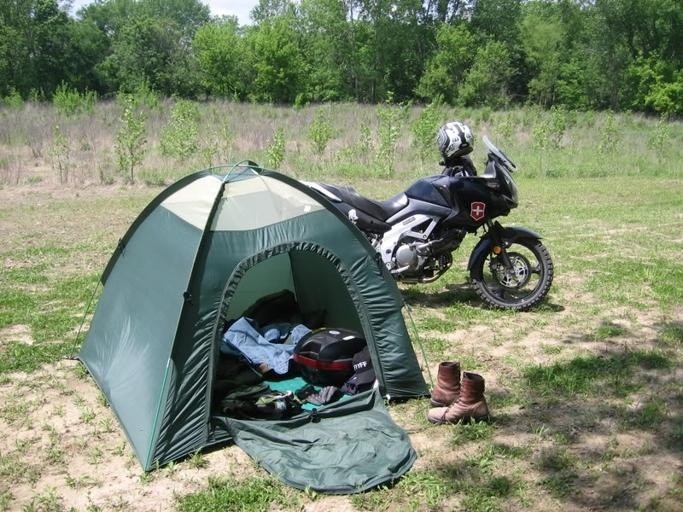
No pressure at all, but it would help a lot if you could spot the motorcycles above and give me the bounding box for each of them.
[300,136,554,311]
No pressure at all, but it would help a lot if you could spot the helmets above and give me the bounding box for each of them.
[435,120,475,161]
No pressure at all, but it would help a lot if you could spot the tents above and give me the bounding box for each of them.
[69,157,434,496]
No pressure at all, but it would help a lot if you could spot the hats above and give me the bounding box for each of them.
[353,349,378,387]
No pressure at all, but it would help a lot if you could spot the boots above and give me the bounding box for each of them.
[429,359,464,410]
[425,369,490,427]
